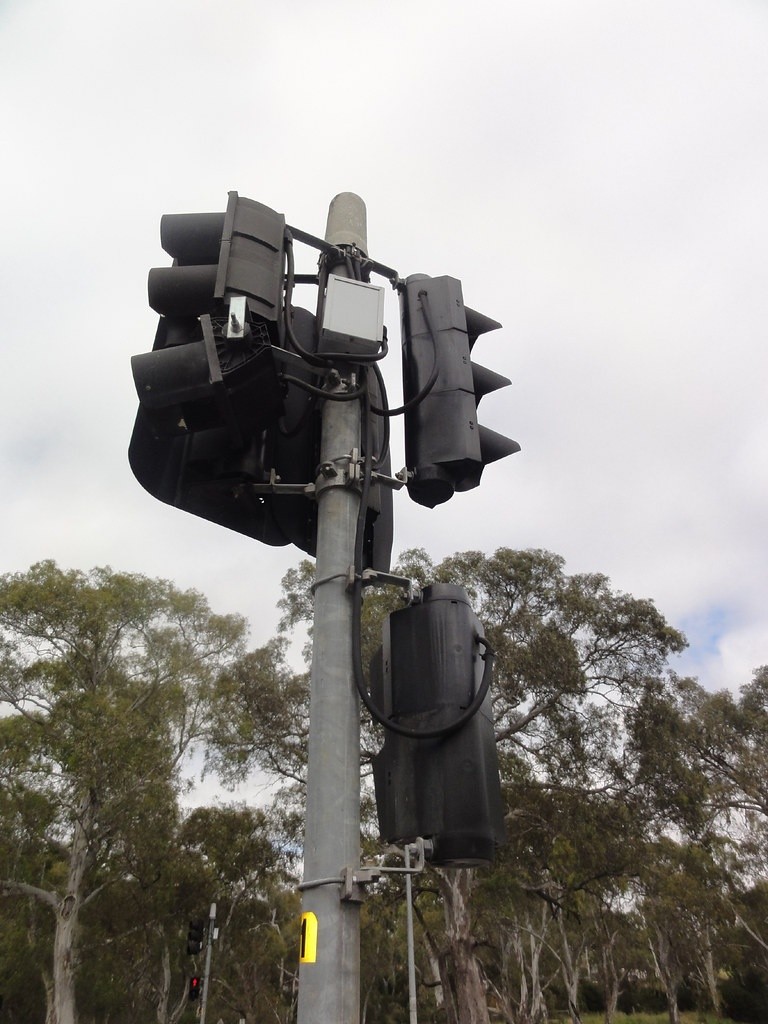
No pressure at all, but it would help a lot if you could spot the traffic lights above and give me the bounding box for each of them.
[186,918,205,955]
[188,976,201,1003]
[144,187,289,344]
[399,269,523,511]
[125,318,286,436]
